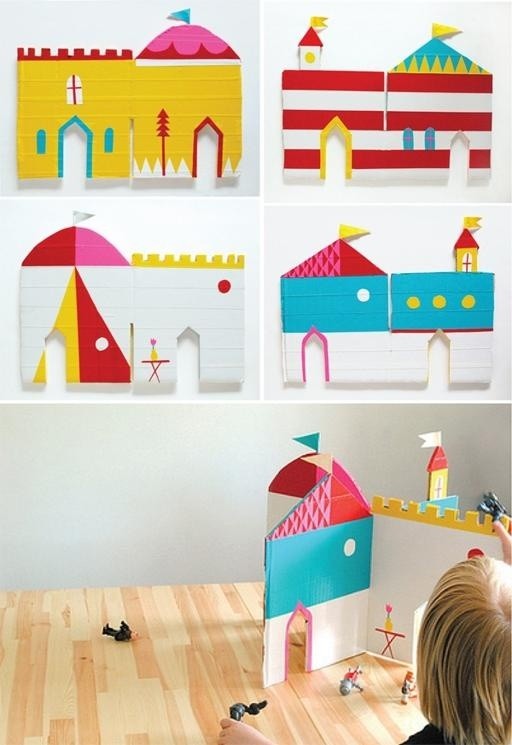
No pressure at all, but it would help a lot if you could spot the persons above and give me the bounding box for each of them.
[340,661,365,697]
[216,518,511,744]
[102,619,142,641]
[228,699,267,721]
[476,491,507,523]
[400,669,418,705]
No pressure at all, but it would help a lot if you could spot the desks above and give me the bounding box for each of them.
[1,583,429,745]
